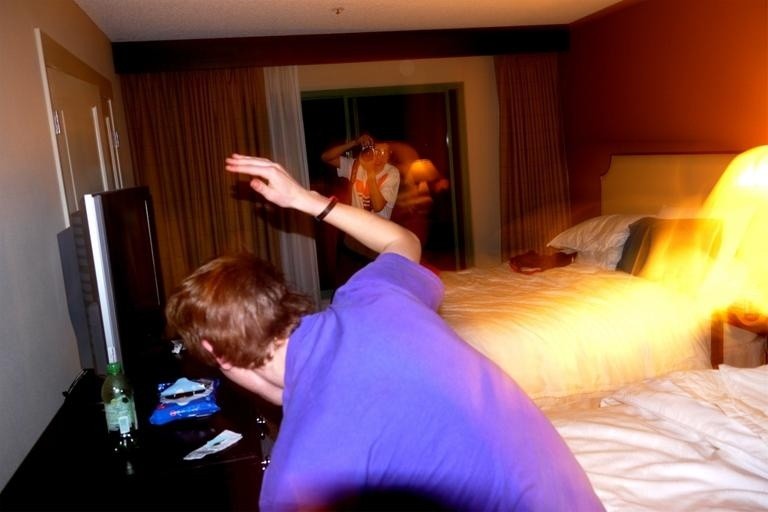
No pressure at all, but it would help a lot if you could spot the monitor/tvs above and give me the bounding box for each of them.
[57,185,170,384]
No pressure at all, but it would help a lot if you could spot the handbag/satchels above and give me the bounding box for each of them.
[508,246,580,275]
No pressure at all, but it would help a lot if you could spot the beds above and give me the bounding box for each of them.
[543,363,768,512]
[438,152,768,409]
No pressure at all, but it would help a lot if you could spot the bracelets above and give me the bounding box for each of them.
[315,195,341,222]
[354,135,360,145]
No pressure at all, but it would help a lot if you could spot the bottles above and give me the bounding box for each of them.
[101,362,139,454]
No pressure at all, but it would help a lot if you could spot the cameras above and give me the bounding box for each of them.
[361,144,375,162]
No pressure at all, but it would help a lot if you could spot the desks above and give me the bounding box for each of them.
[0,337,264,512]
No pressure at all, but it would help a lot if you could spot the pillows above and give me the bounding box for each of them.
[547,214,657,255]
[570,245,624,271]
[615,217,723,284]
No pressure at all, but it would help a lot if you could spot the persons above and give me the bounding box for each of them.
[165,151,610,511]
[321,134,402,304]
